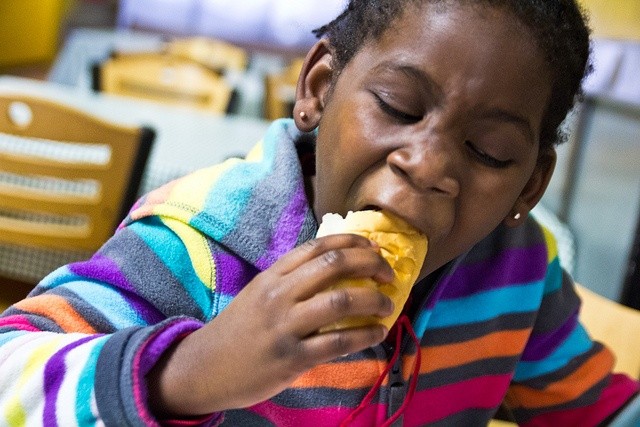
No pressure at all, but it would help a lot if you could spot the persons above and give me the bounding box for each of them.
[0,0,640,426]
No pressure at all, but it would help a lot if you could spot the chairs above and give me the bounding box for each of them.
[0,31,307,288]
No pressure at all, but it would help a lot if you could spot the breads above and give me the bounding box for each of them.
[314,207,430,357]
